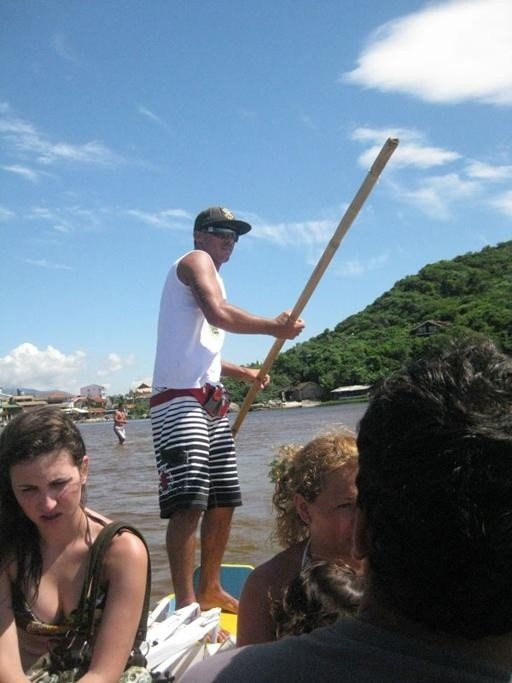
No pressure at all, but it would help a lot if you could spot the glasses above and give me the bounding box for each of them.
[198,227,238,242]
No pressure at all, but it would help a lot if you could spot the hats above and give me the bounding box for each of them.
[194,207,251,235]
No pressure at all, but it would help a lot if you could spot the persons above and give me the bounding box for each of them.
[176,332,512,682]
[235,422,377,647]
[266,558,370,643]
[113,402,129,445]
[147,205,306,646]
[0,404,157,683]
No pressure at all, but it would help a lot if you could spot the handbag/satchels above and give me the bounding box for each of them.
[28,633,152,683]
